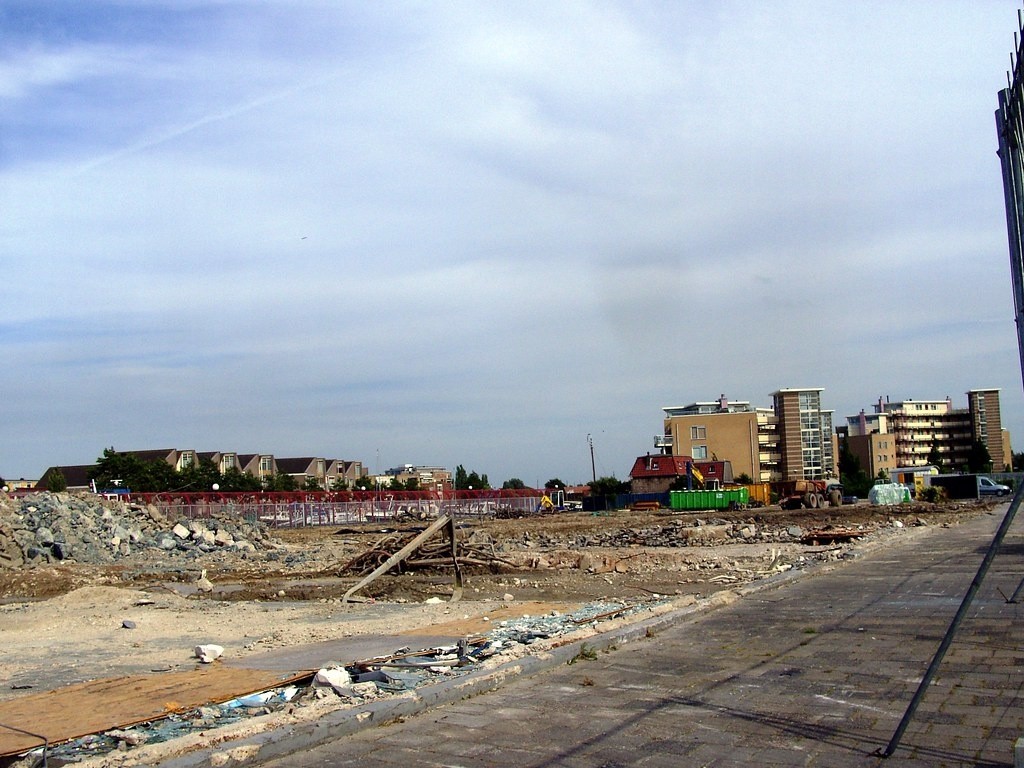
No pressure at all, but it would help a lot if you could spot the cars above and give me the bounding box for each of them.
[977,476,1011,497]
[262,497,511,526]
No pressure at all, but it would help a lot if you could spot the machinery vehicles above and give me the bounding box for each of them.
[778,476,845,510]
